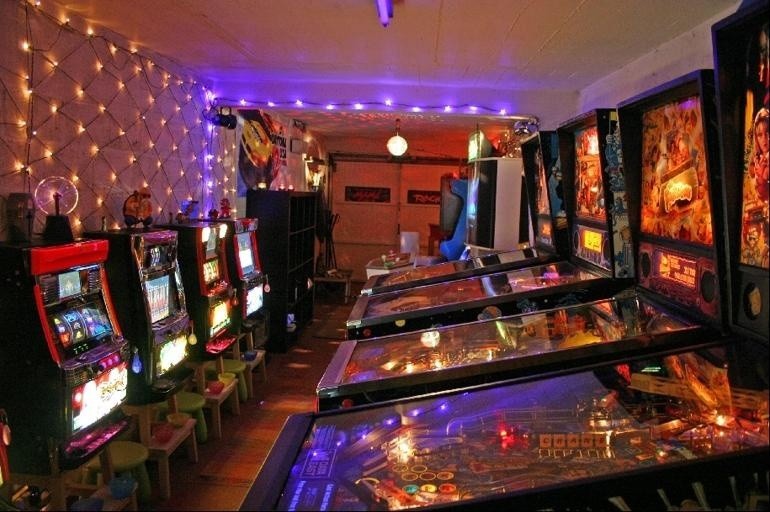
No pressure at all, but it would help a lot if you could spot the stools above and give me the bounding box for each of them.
[76,358,249,505]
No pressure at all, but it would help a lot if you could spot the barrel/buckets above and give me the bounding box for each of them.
[400,231,421,256]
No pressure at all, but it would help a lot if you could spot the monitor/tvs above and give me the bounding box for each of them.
[204,255,225,289]
[44,292,115,360]
[235,232,259,277]
[144,268,181,325]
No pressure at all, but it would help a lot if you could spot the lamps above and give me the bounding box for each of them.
[386,118,409,156]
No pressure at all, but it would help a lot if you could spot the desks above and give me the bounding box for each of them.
[314,267,354,305]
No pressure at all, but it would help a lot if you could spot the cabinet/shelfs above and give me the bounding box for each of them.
[245,187,319,356]
